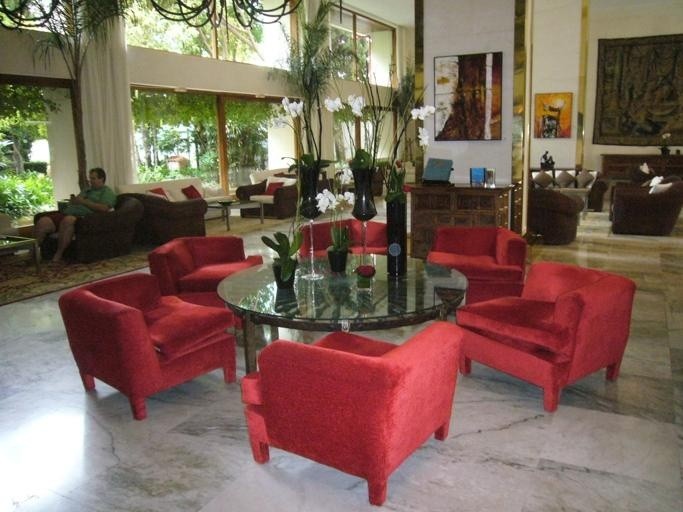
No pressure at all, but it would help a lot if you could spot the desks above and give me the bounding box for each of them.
[206,200,264,230]
[218,252,468,375]
[600,153,683,182]
[0,234,42,274]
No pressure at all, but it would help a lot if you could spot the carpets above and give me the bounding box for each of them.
[0,215,284,305]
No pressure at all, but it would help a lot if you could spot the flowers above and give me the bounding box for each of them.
[260,97,311,281]
[325,94,383,169]
[269,113,333,169]
[660,133,672,149]
[379,106,435,203]
[313,160,355,250]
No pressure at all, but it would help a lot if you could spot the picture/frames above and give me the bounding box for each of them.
[533,93,573,138]
[433,50,503,140]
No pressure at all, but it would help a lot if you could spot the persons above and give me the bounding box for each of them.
[542,150,553,163]
[12,168,118,267]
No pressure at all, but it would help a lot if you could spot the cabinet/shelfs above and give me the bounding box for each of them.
[405,186,510,259]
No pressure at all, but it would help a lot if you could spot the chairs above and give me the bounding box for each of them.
[240,319,465,506]
[118,193,207,244]
[148,235,262,329]
[298,219,389,256]
[427,223,524,304]
[235,174,299,220]
[528,189,584,245]
[34,197,144,263]
[609,175,683,235]
[457,263,636,413]
[59,272,236,421]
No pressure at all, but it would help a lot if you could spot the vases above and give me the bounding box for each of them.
[327,248,348,273]
[271,260,295,289]
[300,167,325,281]
[352,166,377,276]
[662,149,670,155]
[386,202,407,276]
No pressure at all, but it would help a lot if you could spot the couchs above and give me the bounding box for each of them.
[529,169,608,213]
[117,178,231,220]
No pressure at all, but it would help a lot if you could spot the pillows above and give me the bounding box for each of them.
[264,176,297,196]
[144,185,203,201]
[532,170,597,189]
